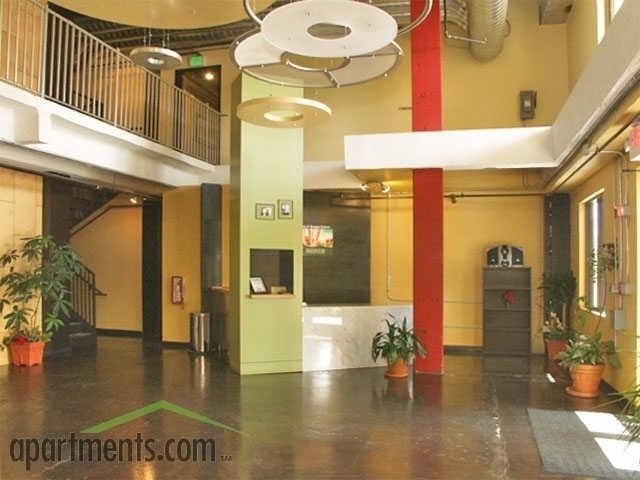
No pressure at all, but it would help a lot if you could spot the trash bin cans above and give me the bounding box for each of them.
[191,313,211,353]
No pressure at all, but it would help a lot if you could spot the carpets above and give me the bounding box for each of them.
[525,407,640,480]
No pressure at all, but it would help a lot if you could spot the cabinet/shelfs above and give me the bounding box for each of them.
[482,266,532,357]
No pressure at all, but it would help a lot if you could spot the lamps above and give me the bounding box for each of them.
[359,180,391,195]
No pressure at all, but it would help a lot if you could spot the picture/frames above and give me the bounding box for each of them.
[171,275,185,305]
[254,199,294,221]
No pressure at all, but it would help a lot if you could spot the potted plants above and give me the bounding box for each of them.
[536,244,622,399]
[371,313,428,379]
[0,234,85,368]
